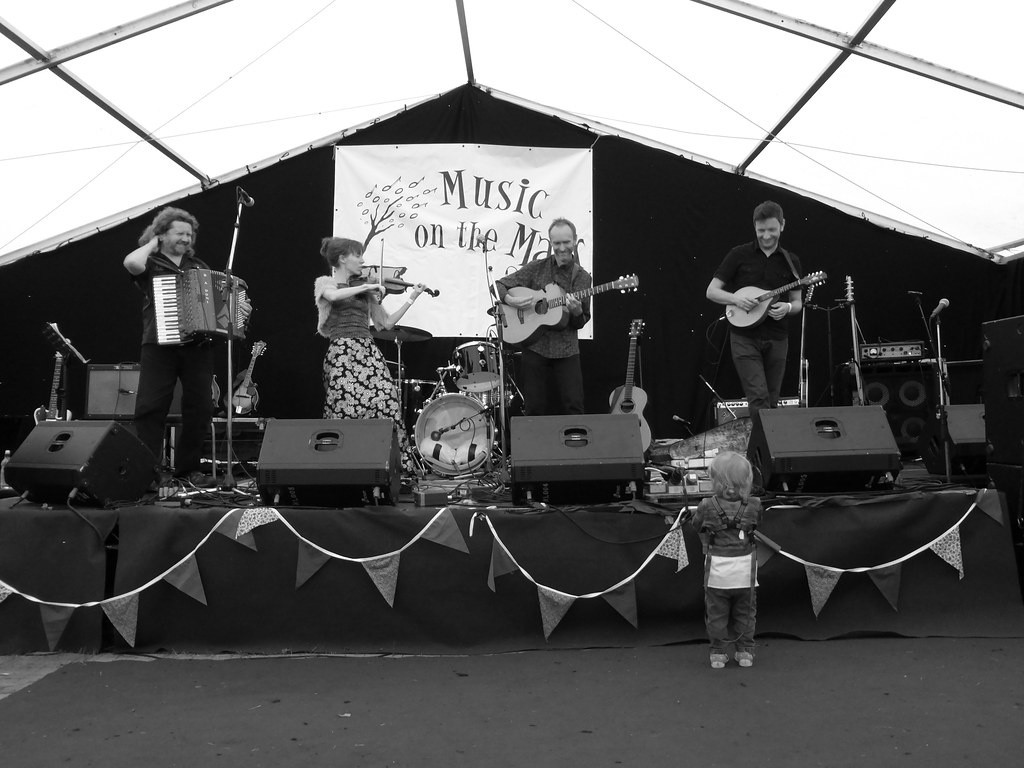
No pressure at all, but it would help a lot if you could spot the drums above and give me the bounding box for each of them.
[460,382,514,408]
[450,341,500,393]
[393,378,442,452]
[414,393,496,476]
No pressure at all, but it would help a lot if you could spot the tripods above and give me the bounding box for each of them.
[177,204,261,506]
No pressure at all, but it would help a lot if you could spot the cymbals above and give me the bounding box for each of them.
[370,325,433,342]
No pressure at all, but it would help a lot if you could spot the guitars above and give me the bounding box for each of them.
[33,337,72,427]
[725,270,828,329]
[225,340,268,414]
[843,275,865,409]
[501,273,640,348]
[608,320,656,456]
[798,284,815,408]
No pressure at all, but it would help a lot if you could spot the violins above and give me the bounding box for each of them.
[348,275,441,298]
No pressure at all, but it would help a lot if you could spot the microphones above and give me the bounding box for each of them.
[238,186,255,207]
[119,388,135,394]
[930,298,950,318]
[475,233,486,245]
[673,415,691,424]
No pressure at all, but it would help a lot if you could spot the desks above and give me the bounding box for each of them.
[162,418,259,480]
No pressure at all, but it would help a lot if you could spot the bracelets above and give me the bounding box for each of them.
[788,303,792,313]
[406,297,413,305]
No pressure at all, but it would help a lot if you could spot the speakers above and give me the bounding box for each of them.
[746,404,902,494]
[3,419,159,508]
[714,397,802,428]
[986,455,1024,610]
[84,364,183,416]
[922,403,985,474]
[981,315,1024,466]
[510,412,645,506]
[256,418,403,507]
[858,363,936,459]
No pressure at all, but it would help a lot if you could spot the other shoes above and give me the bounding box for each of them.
[709,653,729,668]
[172,471,216,488]
[734,651,754,667]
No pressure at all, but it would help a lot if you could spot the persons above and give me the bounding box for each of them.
[313,237,423,478]
[489,218,592,416]
[705,200,803,426]
[691,451,764,668]
[124,206,213,499]
